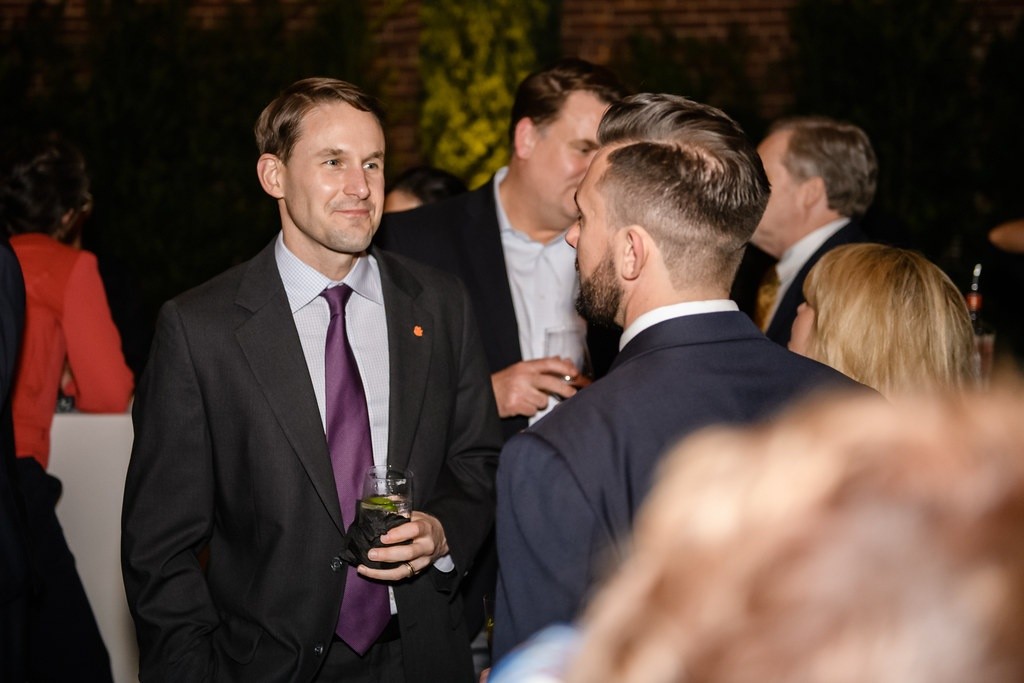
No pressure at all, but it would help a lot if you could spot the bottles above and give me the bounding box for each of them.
[965,264,984,329]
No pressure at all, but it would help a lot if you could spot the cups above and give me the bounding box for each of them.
[543,323,592,403]
[354,463,414,572]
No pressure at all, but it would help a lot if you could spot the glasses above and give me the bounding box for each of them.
[81,193,92,211]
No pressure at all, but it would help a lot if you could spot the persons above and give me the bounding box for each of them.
[119,72,497,683]
[482,88,889,683]
[365,58,613,683]
[785,245,983,412]
[740,117,874,356]
[377,165,471,216]
[545,377,1024,683]
[1,138,136,683]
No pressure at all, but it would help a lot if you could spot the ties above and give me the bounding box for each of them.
[752,266,782,333]
[319,282,391,659]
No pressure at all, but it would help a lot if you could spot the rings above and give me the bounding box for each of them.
[404,563,416,577]
